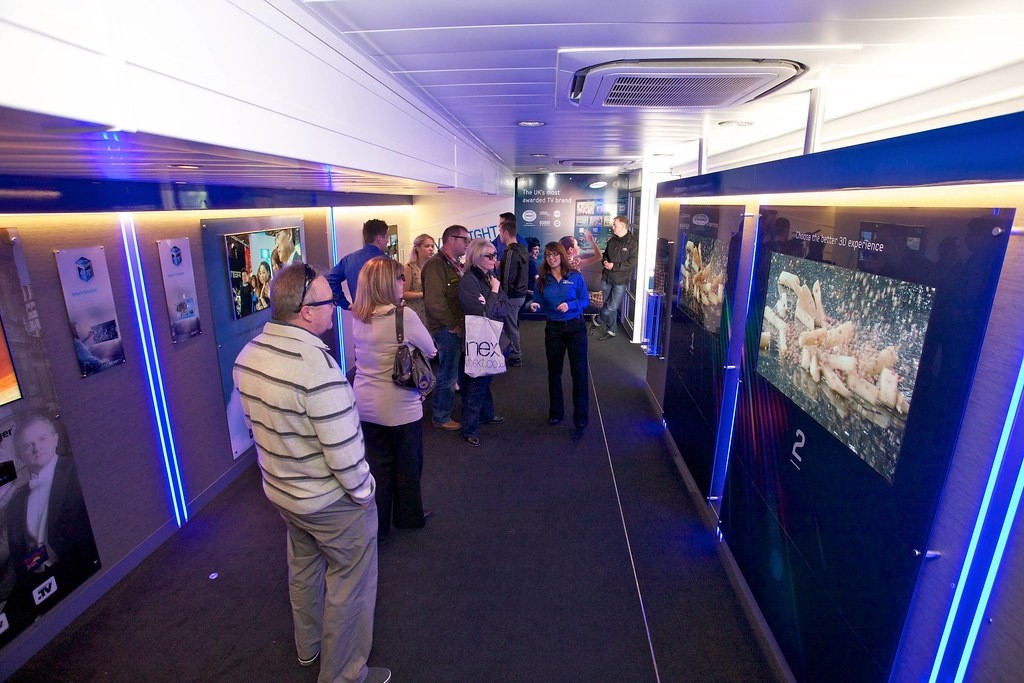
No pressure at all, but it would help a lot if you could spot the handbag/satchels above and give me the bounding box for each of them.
[464,313,507,378]
[392,306,437,396]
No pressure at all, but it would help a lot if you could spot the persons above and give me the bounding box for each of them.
[491,212,528,282]
[232,228,302,320]
[457,238,513,447]
[4,414,101,621]
[70,321,109,370]
[525,237,540,304]
[421,225,472,431]
[353,256,433,531]
[559,229,603,273]
[530,241,590,441]
[588,216,638,341]
[232,262,392,683]
[327,219,391,362]
[403,234,435,333]
[498,220,529,368]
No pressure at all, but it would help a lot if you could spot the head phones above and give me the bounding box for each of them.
[438,249,462,266]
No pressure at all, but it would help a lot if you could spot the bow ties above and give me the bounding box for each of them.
[28,477,49,489]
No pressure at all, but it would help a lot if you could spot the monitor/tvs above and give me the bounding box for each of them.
[755,250,938,486]
[676,232,733,343]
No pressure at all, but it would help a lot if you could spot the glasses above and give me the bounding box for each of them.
[396,273,405,283]
[546,251,559,258]
[480,252,497,260]
[293,264,316,313]
[296,293,339,312]
[447,235,470,243]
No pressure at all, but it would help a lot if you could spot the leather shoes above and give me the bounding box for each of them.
[434,419,461,431]
[478,416,505,424]
[464,437,480,447]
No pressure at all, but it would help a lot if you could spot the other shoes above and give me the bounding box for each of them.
[455,385,461,393]
[507,343,515,353]
[548,415,563,425]
[507,357,521,367]
[574,421,586,429]
[588,318,597,336]
[423,508,432,520]
[597,332,615,341]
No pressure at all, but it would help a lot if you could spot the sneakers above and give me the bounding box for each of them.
[361,666,392,683]
[298,648,321,667]
